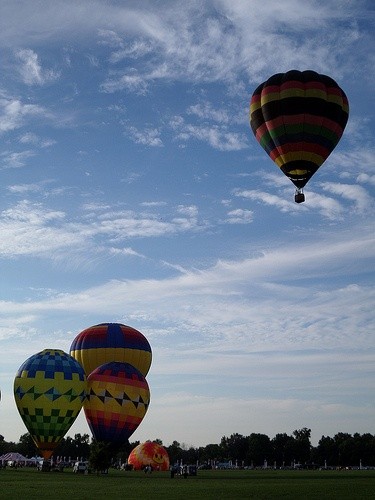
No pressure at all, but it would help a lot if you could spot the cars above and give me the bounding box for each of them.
[37,460,51,472]
[73,462,85,472]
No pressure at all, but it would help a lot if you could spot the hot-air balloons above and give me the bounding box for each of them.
[69,323,151,378]
[127,441,169,470]
[83,362,152,437]
[15,348,87,472]
[249,70,349,203]
